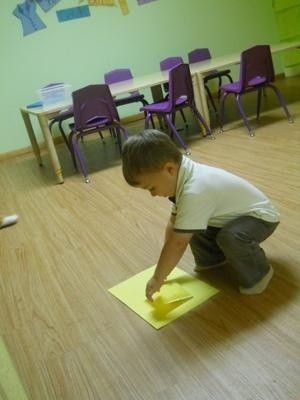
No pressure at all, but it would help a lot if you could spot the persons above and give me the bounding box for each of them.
[121,129,279,302]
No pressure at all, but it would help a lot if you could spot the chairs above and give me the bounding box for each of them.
[104,67,158,139]
[160,54,201,131]
[42,82,129,191]
[140,63,216,156]
[219,41,294,137]
[186,47,239,113]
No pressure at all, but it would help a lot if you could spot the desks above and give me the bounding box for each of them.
[19,40,300,185]
